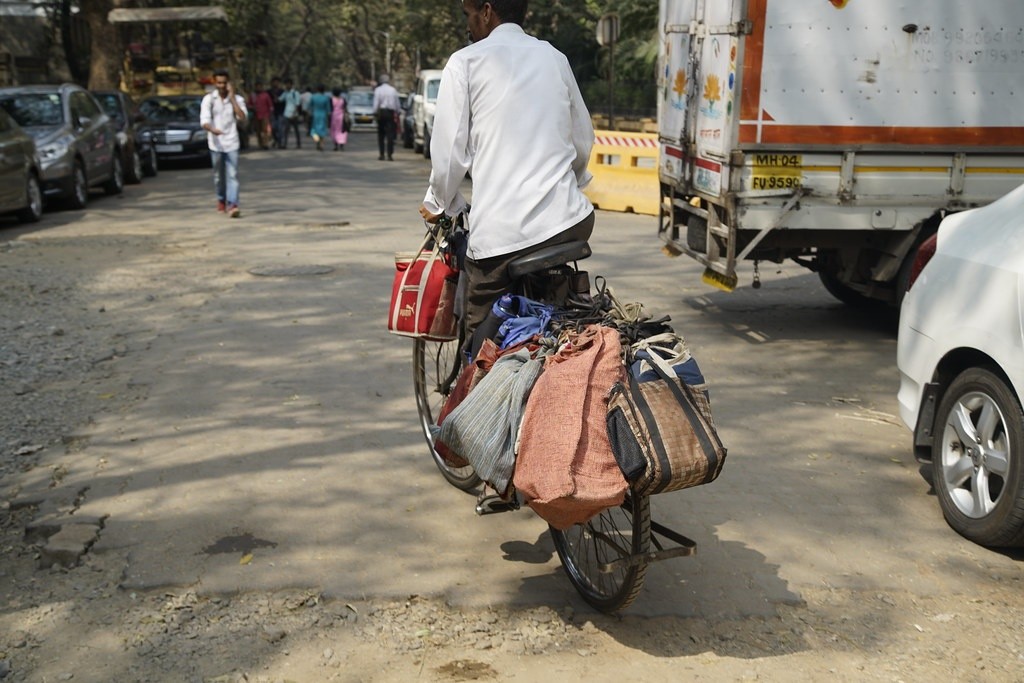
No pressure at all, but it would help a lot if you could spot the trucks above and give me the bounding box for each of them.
[655,0,1024,321]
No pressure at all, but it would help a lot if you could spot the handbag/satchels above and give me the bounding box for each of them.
[429,323,727,532]
[387,216,467,342]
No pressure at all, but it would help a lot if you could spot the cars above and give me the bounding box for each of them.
[137,94,214,169]
[87,89,159,185]
[896,180,1024,551]
[0,106,46,224]
[0,82,124,210]
[344,67,446,158]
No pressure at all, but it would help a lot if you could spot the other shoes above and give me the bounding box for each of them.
[316,142,323,150]
[334,144,344,151]
[379,155,393,161]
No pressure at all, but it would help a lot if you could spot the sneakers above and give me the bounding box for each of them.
[228,204,240,217]
[217,201,225,214]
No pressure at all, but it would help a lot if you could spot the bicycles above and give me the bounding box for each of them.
[410,202,696,615]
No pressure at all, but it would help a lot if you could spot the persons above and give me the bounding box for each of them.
[199,70,250,218]
[328,86,351,151]
[307,84,334,151]
[372,74,402,162]
[265,76,287,149]
[299,86,314,137]
[274,78,302,150]
[247,80,276,151]
[420,0,596,518]
